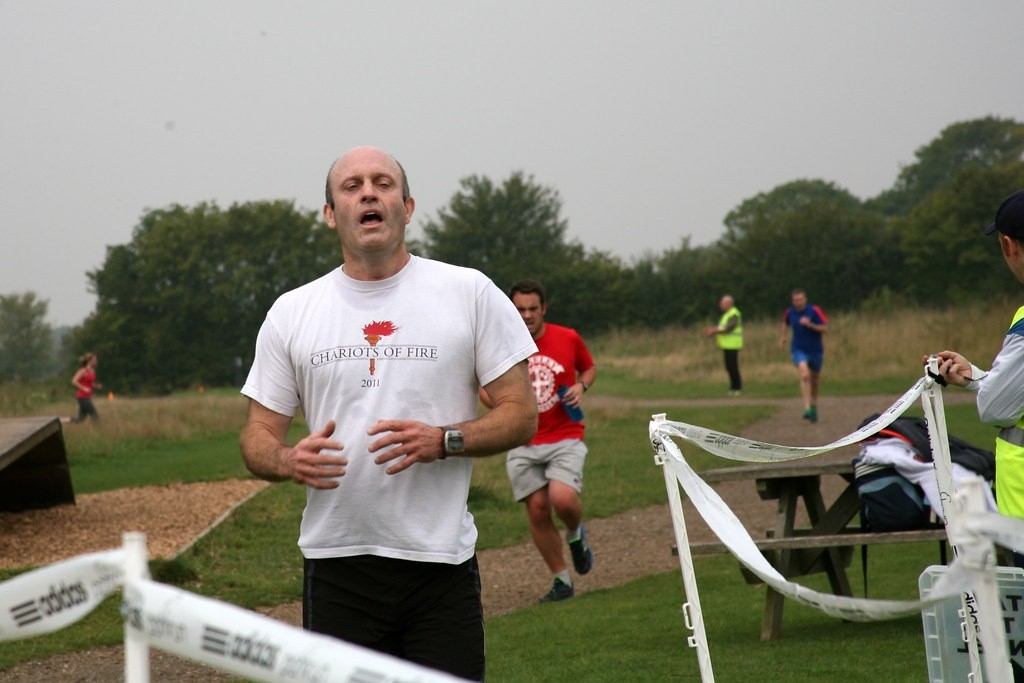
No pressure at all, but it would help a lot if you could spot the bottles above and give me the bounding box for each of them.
[558,383,584,422]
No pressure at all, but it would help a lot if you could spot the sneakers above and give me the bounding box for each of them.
[565,523,593,575]
[538,577,574,603]
[811,413,816,421]
[803,411,812,419]
[726,389,744,398]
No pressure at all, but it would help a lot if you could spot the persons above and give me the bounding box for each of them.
[61,350,104,424]
[922,191,1023,682]
[706,296,744,398]
[780,289,829,424]
[238,147,540,683]
[481,283,601,605]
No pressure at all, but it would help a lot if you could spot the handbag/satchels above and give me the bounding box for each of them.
[852,458,932,534]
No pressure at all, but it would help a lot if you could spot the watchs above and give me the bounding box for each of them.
[576,380,588,392]
[436,425,465,462]
[927,355,989,388]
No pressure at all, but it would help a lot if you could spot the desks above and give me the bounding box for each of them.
[671,455,1015,641]
